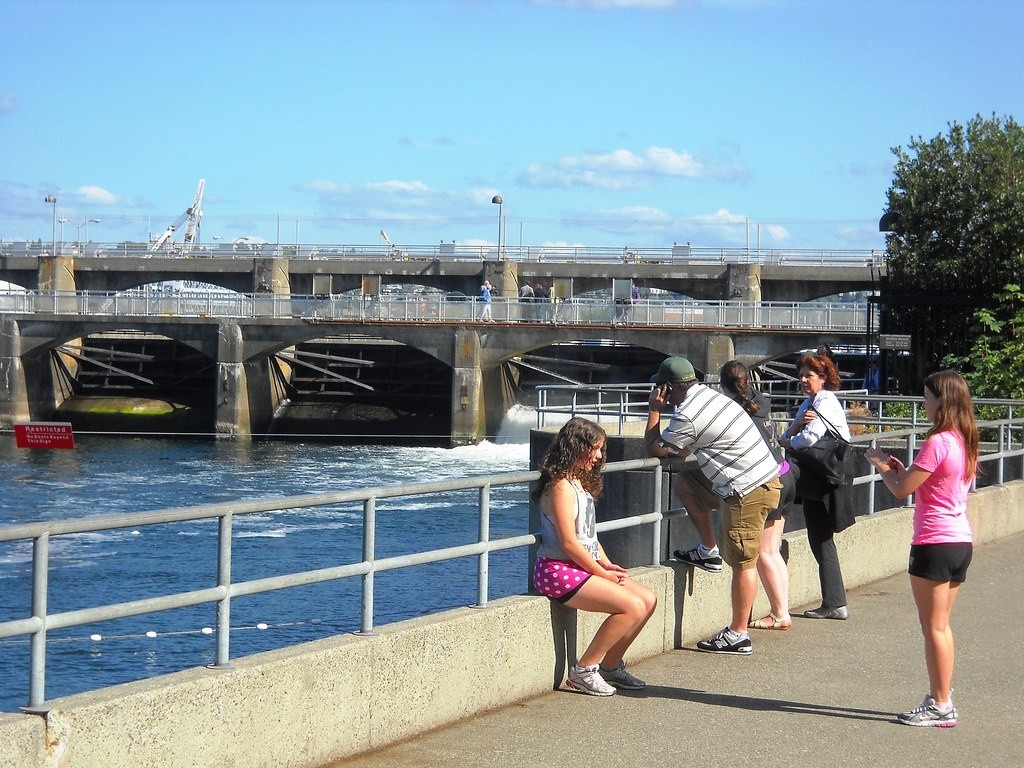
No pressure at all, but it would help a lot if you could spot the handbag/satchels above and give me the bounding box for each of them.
[785,405,851,494]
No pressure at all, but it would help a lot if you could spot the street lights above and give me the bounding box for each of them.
[45,195,57,256]
[59,219,101,255]
[492,195,504,261]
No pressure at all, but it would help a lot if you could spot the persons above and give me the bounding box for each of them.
[720,361,796,630]
[475,281,549,323]
[780,355,855,620]
[532,417,658,697]
[632,283,639,303]
[863,370,980,728]
[646,357,785,655]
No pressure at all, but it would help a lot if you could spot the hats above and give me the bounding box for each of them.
[650,356,696,383]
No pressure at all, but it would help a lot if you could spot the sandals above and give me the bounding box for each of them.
[747,613,791,630]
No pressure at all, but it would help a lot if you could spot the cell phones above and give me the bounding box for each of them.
[660,384,673,397]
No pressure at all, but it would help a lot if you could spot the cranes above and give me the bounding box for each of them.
[145,178,207,260]
[380,230,393,245]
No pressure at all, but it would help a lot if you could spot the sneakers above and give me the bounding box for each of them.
[897,688,959,726]
[674,542,723,573]
[697,627,753,655]
[597,660,645,689]
[566,664,617,696]
[804,606,848,620]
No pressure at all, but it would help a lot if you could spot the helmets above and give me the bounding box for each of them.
[867,355,878,363]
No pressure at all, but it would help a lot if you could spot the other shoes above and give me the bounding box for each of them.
[477,319,484,322]
[488,320,495,323]
